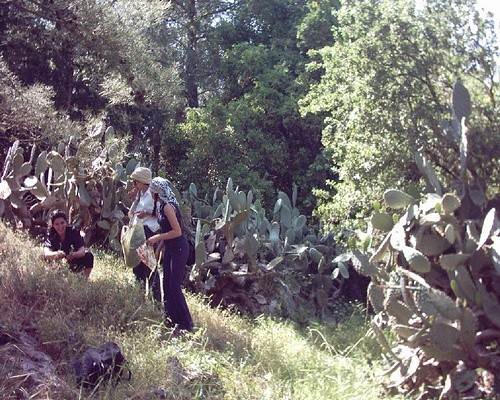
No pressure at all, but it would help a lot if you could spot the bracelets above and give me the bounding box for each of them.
[144,209,146,215]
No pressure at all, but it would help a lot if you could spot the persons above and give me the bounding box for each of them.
[44,213,94,281]
[128,167,161,308]
[148,177,196,333]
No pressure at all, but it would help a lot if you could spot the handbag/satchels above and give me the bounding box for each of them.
[187,240,195,266]
[121,216,146,268]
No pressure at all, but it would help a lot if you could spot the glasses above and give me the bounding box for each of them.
[133,181,140,185]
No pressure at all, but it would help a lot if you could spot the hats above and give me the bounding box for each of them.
[130,167,151,184]
[151,177,168,194]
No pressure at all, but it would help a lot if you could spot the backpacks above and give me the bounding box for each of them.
[75,342,125,390]
